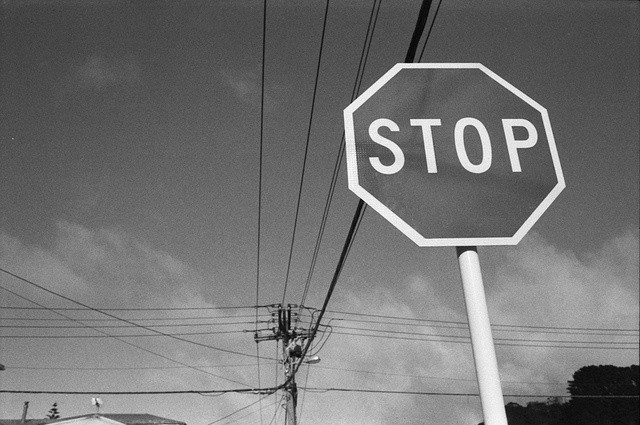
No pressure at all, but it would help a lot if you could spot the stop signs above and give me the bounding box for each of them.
[343,63,566,247]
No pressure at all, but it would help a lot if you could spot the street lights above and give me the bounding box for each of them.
[286,356,320,425]
